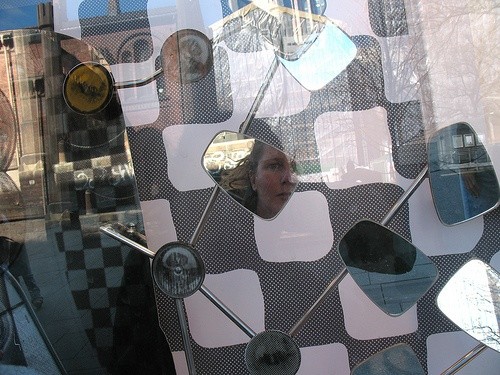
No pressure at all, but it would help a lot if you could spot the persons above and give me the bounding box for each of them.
[216,139,300,220]
